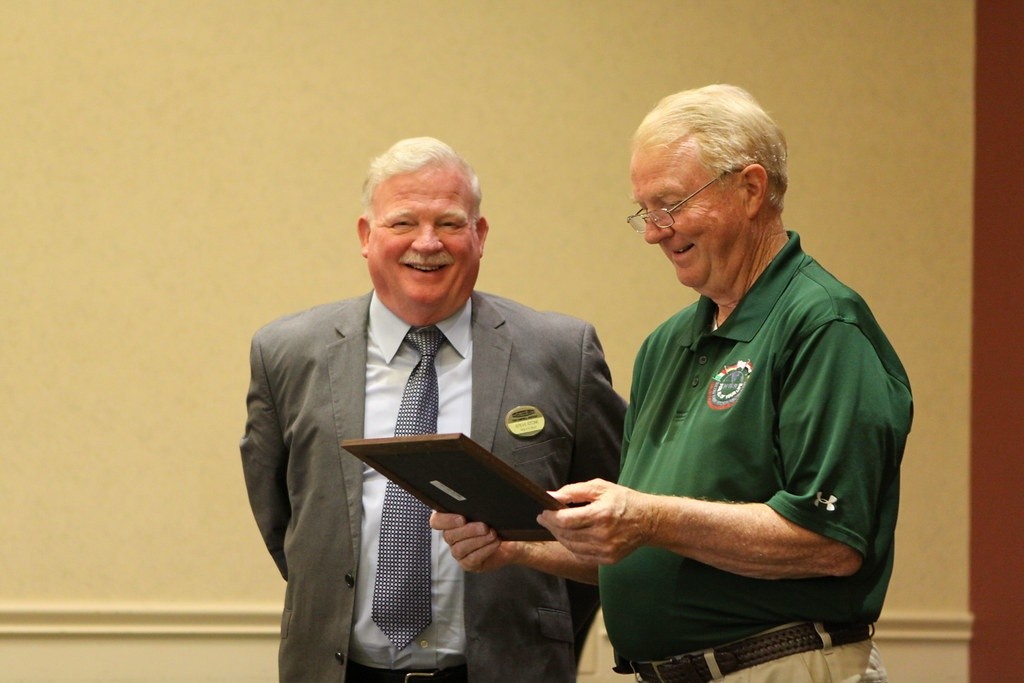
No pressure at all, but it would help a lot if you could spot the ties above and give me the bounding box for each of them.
[370,326,446,652]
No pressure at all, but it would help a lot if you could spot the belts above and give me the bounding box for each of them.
[346,658,469,683]
[613,620,870,683]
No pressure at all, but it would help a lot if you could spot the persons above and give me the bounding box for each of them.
[241,137,627,683]
[429,83,915,683]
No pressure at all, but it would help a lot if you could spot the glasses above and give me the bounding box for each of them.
[627,168,742,233]
